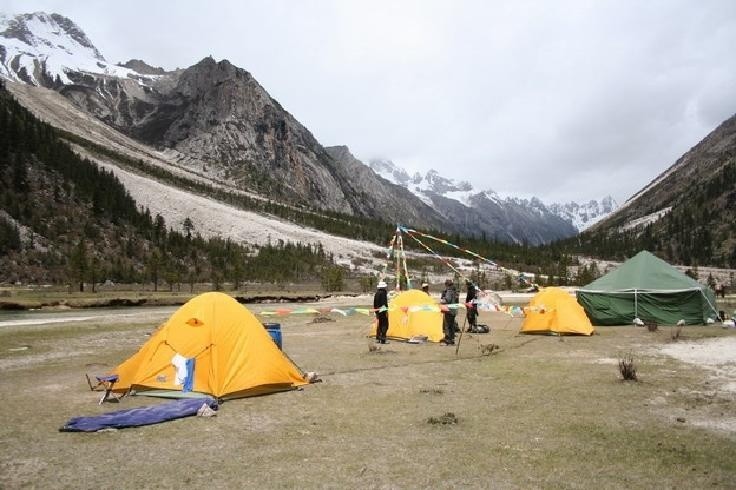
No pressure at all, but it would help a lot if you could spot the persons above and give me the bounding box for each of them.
[372,280,388,345]
[466,279,478,333]
[420,282,428,295]
[439,277,459,345]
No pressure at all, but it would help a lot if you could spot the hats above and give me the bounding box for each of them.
[377,282,388,288]
[422,283,428,288]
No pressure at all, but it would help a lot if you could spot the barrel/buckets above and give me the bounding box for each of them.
[262,322,282,351]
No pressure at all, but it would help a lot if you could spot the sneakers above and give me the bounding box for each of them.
[378,338,457,346]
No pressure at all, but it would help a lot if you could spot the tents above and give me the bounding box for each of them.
[517,286,594,337]
[365,288,444,344]
[575,250,718,327]
[90,292,320,401]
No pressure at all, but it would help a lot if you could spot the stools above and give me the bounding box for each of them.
[96,374,122,404]
